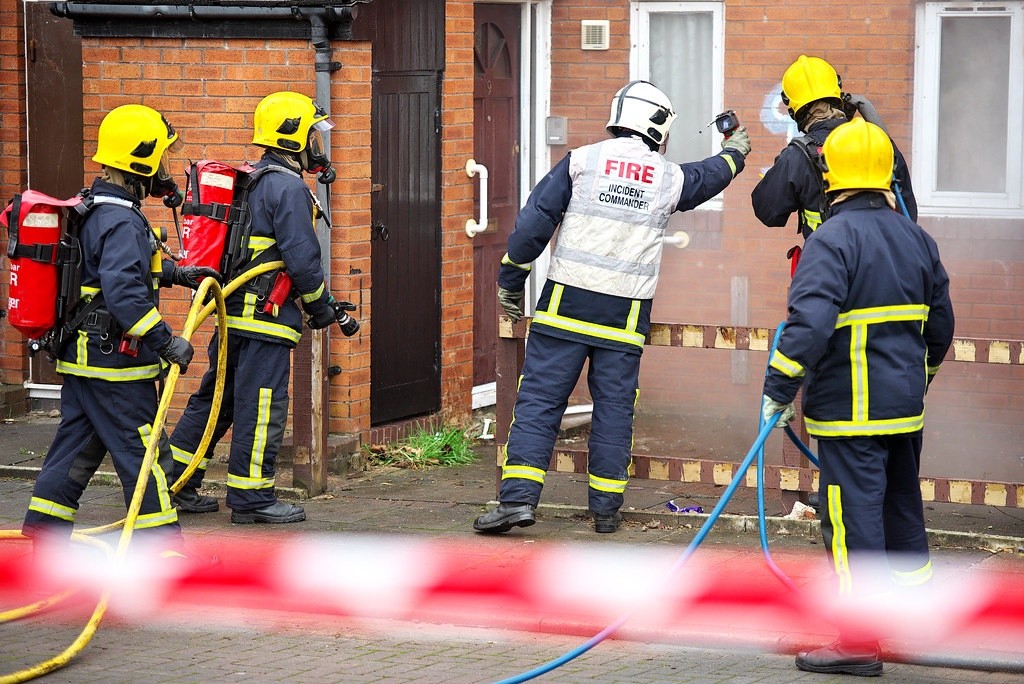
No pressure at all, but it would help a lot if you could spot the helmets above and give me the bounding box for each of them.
[780,55,844,122]
[816,117,894,194]
[251,91,336,158]
[92,103,186,181]
[605,80,678,156]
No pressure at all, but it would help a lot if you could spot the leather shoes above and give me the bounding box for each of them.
[592,510,623,533]
[794,637,884,677]
[168,482,219,512]
[473,502,536,532]
[231,501,306,524]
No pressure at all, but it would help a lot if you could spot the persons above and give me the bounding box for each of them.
[747,56,956,677]
[165,90,334,523]
[20,103,196,570]
[474,81,752,535]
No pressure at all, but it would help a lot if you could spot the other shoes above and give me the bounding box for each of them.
[163,536,224,575]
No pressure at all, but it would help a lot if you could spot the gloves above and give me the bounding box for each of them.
[763,394,796,428]
[313,305,336,328]
[721,125,752,159]
[498,289,525,323]
[159,336,194,375]
